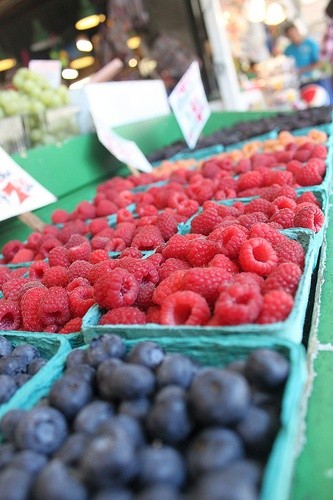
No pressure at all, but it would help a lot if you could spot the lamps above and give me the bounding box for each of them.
[73,0,100,29]
[1,46,16,72]
[66,41,94,70]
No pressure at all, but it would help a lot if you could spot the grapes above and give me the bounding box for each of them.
[0,66,68,142]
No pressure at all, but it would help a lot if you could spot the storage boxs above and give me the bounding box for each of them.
[1,125,330,500]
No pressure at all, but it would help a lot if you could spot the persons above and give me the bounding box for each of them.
[319,1,333,69]
[282,25,322,82]
